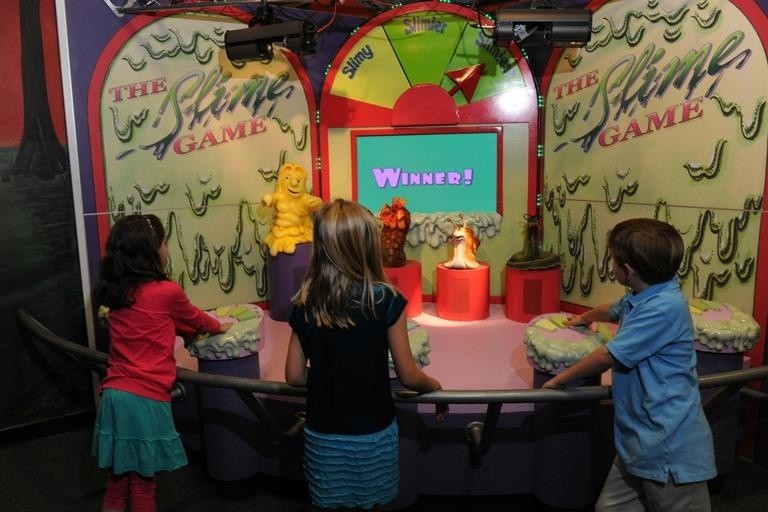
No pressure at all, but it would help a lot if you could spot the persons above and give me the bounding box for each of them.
[542,215,718,511]
[274,198,451,510]
[86,214,234,511]
